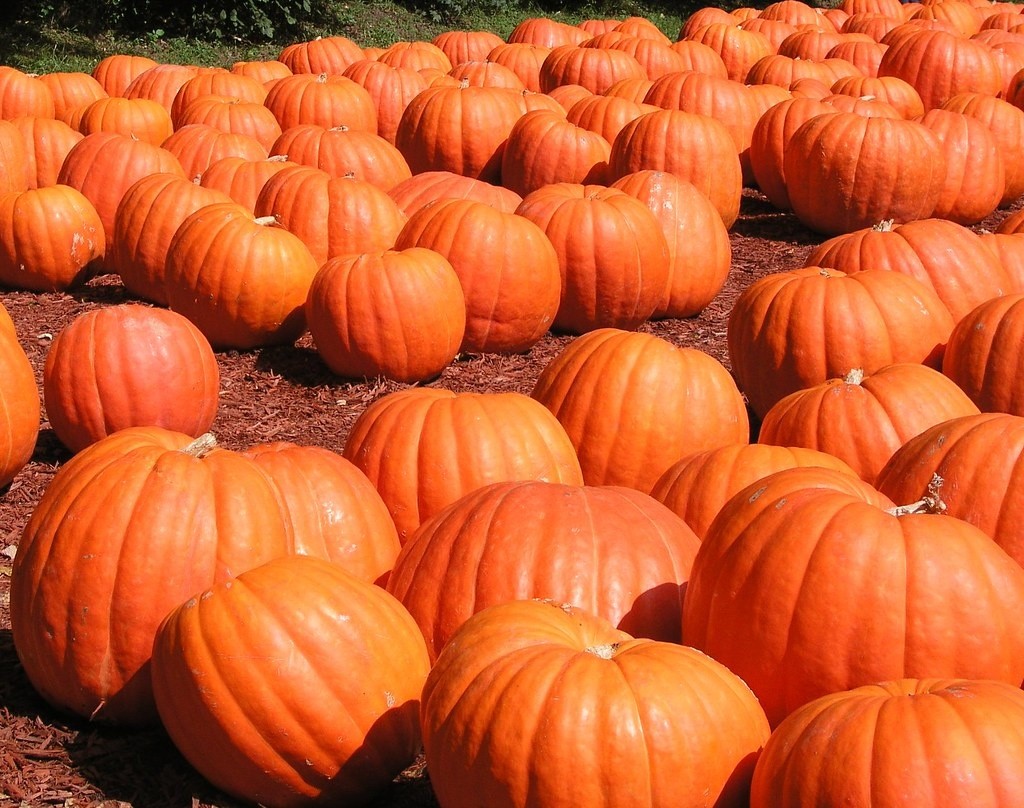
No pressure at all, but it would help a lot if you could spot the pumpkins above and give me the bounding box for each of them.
[0,0,1023,808]
